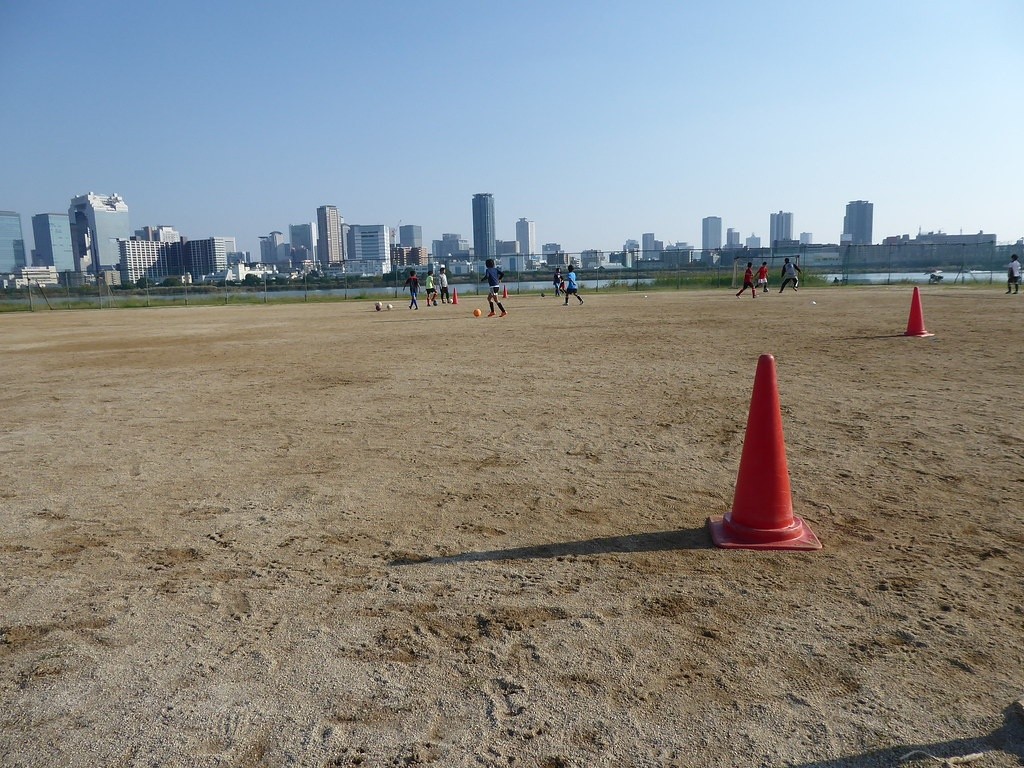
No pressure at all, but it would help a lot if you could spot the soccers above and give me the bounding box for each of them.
[474,309,481,317]
[448,299,453,303]
[375,302,382,311]
[763,288,769,292]
[387,304,392,310]
[541,293,545,297]
[433,301,438,306]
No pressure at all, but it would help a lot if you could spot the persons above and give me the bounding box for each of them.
[834,277,839,282]
[553,267,567,297]
[561,265,584,306]
[1005,254,1021,295]
[778,258,801,293]
[480,259,507,317]
[439,267,452,303]
[754,261,769,292]
[402,271,420,310]
[736,262,759,298]
[425,271,438,307]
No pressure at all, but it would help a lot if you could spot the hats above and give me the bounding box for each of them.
[427,271,433,274]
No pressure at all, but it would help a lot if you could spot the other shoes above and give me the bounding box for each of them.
[793,287,798,291]
[763,290,768,292]
[555,295,561,297]
[562,292,567,294]
[431,299,436,301]
[562,303,568,306]
[579,300,584,305]
[414,308,419,310]
[779,291,782,293]
[409,306,412,309]
[442,301,446,303]
[427,305,431,306]
[447,301,451,303]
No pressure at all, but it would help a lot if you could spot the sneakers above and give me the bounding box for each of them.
[499,312,507,317]
[1012,291,1019,294]
[1005,291,1011,294]
[488,312,496,317]
[736,294,741,299]
[753,295,759,298]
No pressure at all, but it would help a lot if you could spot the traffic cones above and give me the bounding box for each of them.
[706,354,824,552]
[502,285,509,298]
[452,287,459,304]
[903,286,935,337]
